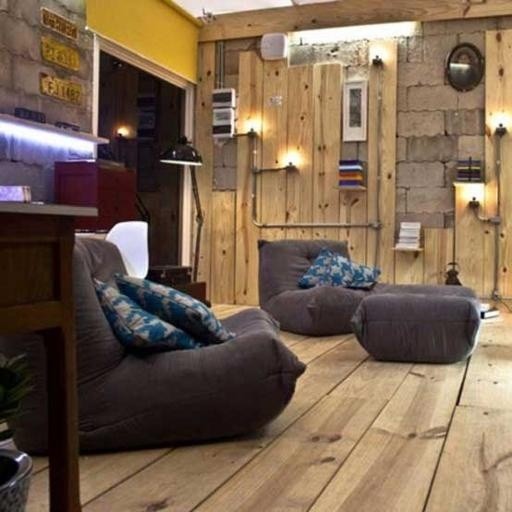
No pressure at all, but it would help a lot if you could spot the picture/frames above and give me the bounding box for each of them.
[446,43,485,94]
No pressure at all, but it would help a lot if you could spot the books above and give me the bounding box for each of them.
[396,222,421,247]
[336,159,363,187]
[479,304,489,312]
[480,308,500,320]
[455,159,482,181]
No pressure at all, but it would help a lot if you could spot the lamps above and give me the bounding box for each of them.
[158,133,207,282]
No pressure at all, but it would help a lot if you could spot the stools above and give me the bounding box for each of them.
[348,282,481,365]
